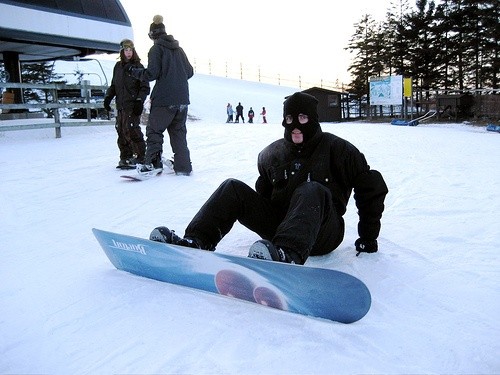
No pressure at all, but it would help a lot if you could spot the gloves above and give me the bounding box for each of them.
[134,100,143,116]
[104,97,110,110]
[355,237,378,253]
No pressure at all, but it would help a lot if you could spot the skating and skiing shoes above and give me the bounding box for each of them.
[150,226,216,251]
[248,240,293,265]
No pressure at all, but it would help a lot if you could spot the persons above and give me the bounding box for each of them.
[226,103,236,123]
[104,39,150,167]
[248,106,254,123]
[260,106,267,123]
[129,15,194,175]
[149,92,388,266]
[236,102,245,123]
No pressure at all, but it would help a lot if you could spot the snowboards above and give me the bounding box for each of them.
[92,227,372,325]
[122,168,160,180]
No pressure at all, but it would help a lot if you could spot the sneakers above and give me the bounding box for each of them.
[137,160,163,177]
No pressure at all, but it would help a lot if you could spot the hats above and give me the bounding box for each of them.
[148,15,165,39]
[120,40,134,49]
